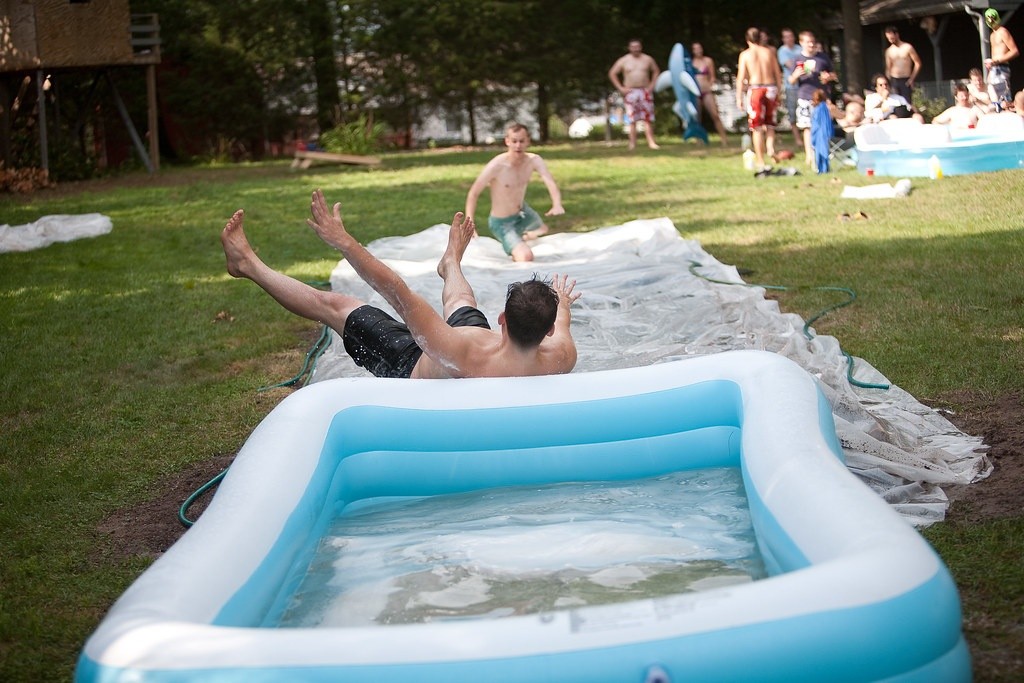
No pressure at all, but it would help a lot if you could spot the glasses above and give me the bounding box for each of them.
[879,82,889,87]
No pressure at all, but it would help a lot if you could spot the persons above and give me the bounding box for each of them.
[221,189,582,378]
[466,124,564,261]
[609,9,1024,170]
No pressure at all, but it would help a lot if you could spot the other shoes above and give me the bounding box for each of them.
[830,174,844,183]
[791,181,820,196]
[841,209,870,225]
